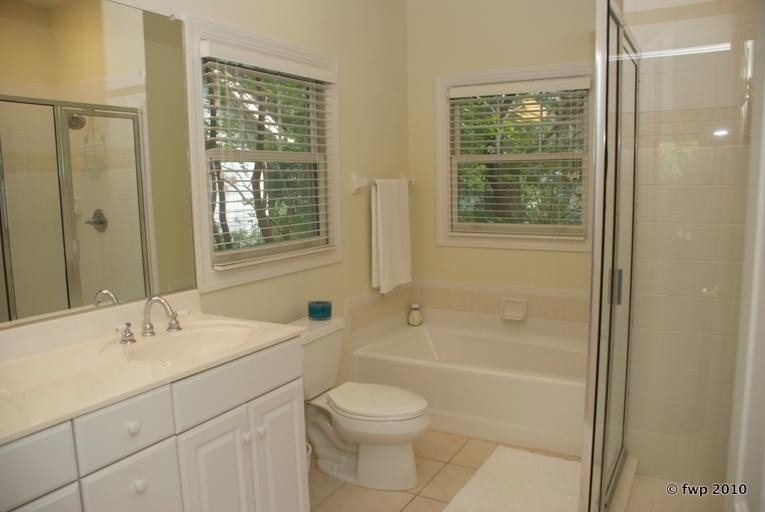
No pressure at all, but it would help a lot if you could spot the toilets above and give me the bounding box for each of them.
[288,315,429,492]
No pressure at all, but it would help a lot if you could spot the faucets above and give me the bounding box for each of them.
[93,288,118,306]
[142,295,182,337]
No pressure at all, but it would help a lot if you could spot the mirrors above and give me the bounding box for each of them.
[1,2,200,329]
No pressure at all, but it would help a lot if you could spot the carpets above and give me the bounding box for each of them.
[443,444,579,512]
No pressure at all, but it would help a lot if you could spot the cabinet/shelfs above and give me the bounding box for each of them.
[0,335,313,511]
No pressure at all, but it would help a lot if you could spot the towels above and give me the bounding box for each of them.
[372,177,414,295]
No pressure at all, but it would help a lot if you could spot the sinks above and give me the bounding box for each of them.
[103,321,259,371]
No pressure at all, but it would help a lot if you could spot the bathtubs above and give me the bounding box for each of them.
[351,321,587,461]
